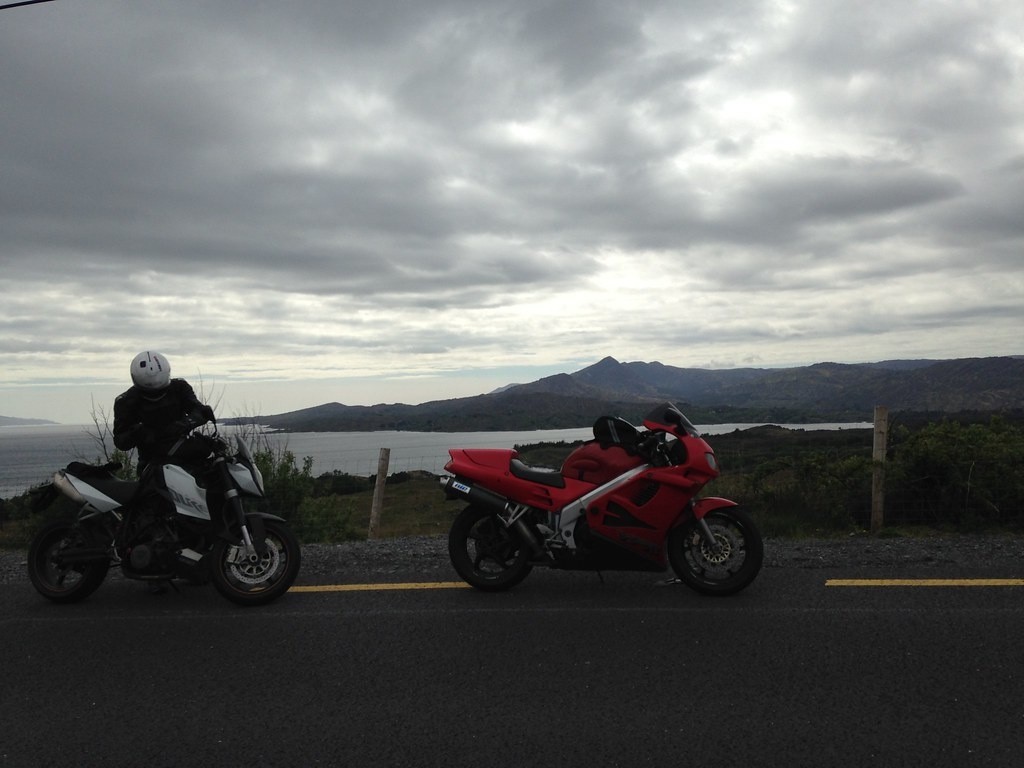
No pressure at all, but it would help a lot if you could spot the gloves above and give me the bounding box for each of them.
[155,420,190,440]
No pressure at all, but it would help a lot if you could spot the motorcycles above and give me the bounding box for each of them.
[437,400,765,595]
[27,422,301,602]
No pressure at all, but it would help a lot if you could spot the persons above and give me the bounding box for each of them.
[113,350,217,593]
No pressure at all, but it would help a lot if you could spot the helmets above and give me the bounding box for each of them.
[132,355,172,402]
